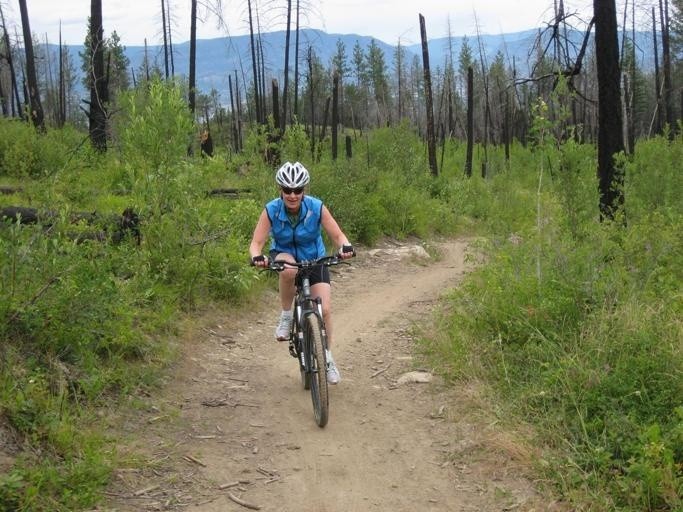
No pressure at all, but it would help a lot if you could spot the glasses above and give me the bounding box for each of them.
[281,185,303,195]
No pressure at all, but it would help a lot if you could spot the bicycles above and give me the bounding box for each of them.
[249,252,357,428]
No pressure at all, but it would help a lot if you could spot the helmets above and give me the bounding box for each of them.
[274,162,310,188]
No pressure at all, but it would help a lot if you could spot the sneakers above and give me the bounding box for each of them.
[275,317,294,340]
[326,361,339,384]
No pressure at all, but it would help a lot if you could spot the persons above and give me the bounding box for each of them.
[248,160,356,386]
